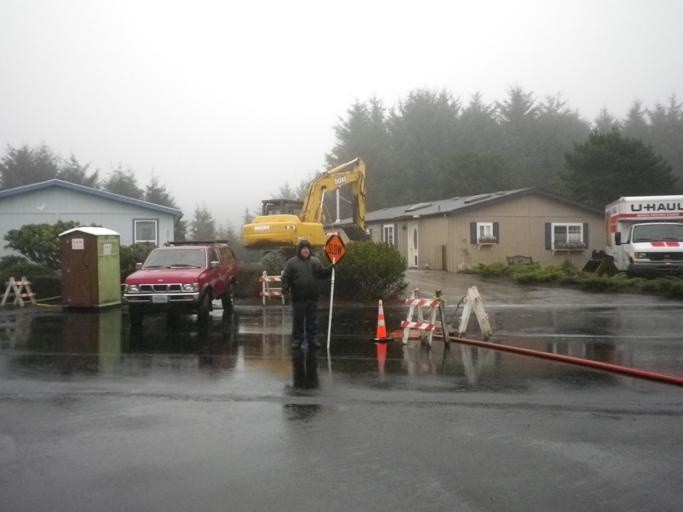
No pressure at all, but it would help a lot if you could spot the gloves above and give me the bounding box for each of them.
[328,263,335,272]
[282,285,289,294]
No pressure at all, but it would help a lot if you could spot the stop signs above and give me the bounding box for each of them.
[322,234,348,266]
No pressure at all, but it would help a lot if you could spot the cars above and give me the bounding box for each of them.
[121,239,242,326]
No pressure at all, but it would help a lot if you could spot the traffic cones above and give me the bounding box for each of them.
[370,298,393,343]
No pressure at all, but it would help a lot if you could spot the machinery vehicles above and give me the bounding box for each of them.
[240,155,371,263]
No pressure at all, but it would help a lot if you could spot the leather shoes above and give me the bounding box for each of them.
[290,342,320,349]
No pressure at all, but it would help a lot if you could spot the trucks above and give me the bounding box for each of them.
[602,193,682,279]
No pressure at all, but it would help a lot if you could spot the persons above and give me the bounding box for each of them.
[280,240,335,349]
